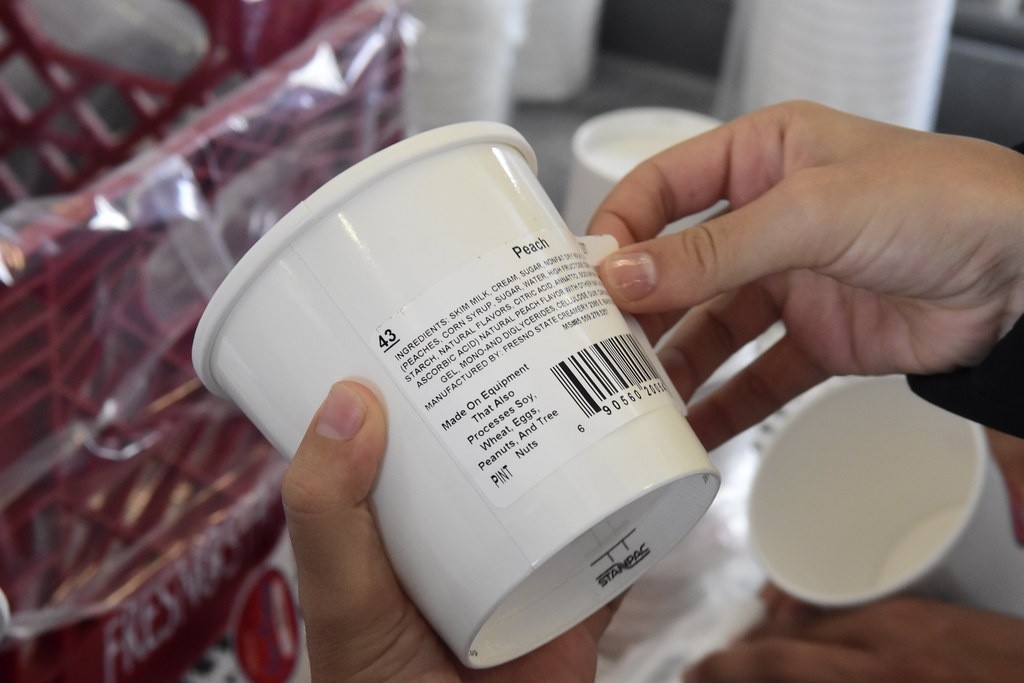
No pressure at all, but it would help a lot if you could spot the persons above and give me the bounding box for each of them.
[278,99,1024,683]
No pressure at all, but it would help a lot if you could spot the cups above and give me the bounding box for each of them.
[375,0,950,158]
[690,326,1024,617]
[565,106,737,252]
[191,120,722,672]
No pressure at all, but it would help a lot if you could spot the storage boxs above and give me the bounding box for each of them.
[0,1,407,683]
